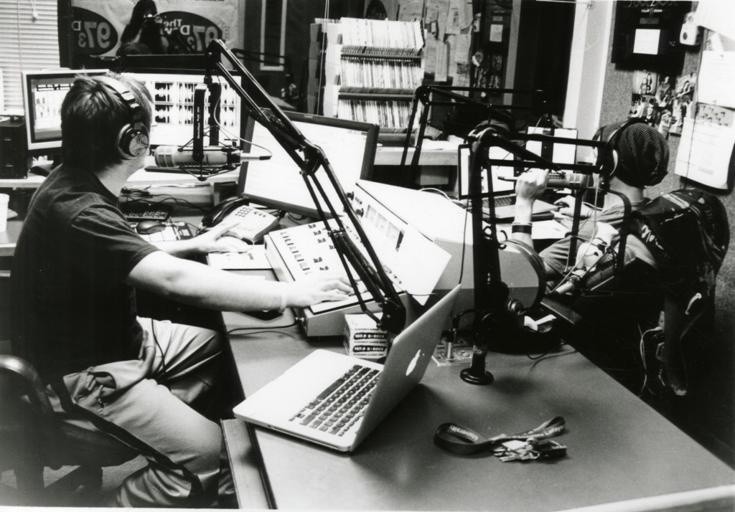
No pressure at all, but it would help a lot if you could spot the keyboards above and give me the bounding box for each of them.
[117,200,171,220]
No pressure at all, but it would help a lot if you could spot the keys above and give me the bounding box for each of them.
[492,437,539,463]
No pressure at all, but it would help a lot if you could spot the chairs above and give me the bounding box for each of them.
[530,184,729,400]
[0,246,221,507]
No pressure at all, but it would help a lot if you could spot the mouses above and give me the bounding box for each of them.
[217,236,249,253]
[137,220,166,235]
[551,202,569,212]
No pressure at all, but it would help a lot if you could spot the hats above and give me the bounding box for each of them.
[600,122,669,186]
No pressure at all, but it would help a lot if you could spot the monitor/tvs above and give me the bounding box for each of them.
[523,125,578,179]
[116,69,248,174]
[237,104,379,219]
[21,68,111,178]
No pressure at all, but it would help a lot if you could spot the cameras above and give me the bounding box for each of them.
[144,14,156,23]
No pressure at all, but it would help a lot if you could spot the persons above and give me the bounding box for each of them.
[10,73,355,507]
[512,118,669,290]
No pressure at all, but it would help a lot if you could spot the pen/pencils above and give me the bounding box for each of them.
[497,176,519,182]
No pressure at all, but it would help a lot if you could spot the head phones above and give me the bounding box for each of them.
[595,116,649,181]
[91,75,150,160]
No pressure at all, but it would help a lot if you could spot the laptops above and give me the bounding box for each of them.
[231,284,463,453]
[458,144,554,224]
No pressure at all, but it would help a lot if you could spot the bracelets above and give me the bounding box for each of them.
[277,280,288,314]
[512,224,533,233]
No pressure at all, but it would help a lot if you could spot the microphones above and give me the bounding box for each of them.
[155,146,270,166]
[499,170,587,190]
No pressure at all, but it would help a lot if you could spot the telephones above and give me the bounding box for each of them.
[201,196,279,243]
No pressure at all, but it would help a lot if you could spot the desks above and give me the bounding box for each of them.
[2,154,317,253]
[205,229,735,512]
[445,184,624,250]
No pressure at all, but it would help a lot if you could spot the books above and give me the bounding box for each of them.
[339,16,423,131]
